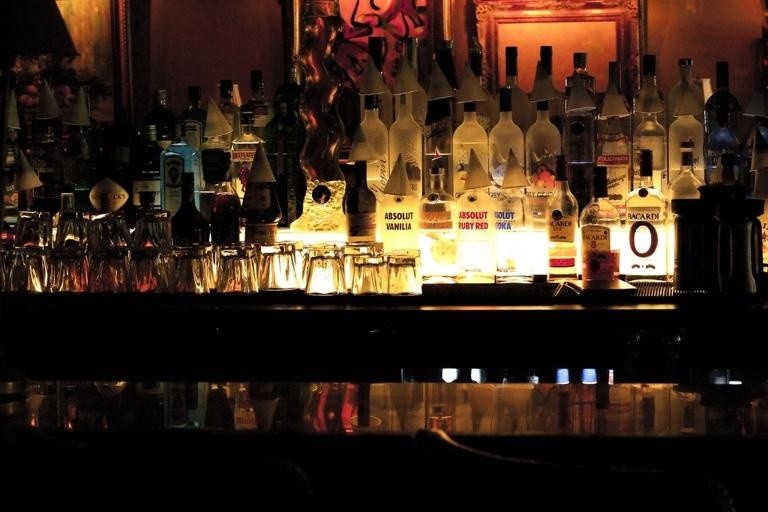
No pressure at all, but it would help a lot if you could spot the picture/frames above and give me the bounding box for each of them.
[290,1,453,93]
[472,0,646,100]
[0,0,137,149]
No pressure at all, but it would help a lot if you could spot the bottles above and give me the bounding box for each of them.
[352,32,759,291]
[0,54,301,244]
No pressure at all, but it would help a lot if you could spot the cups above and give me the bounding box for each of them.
[0,245,352,296]
[0,379,281,427]
